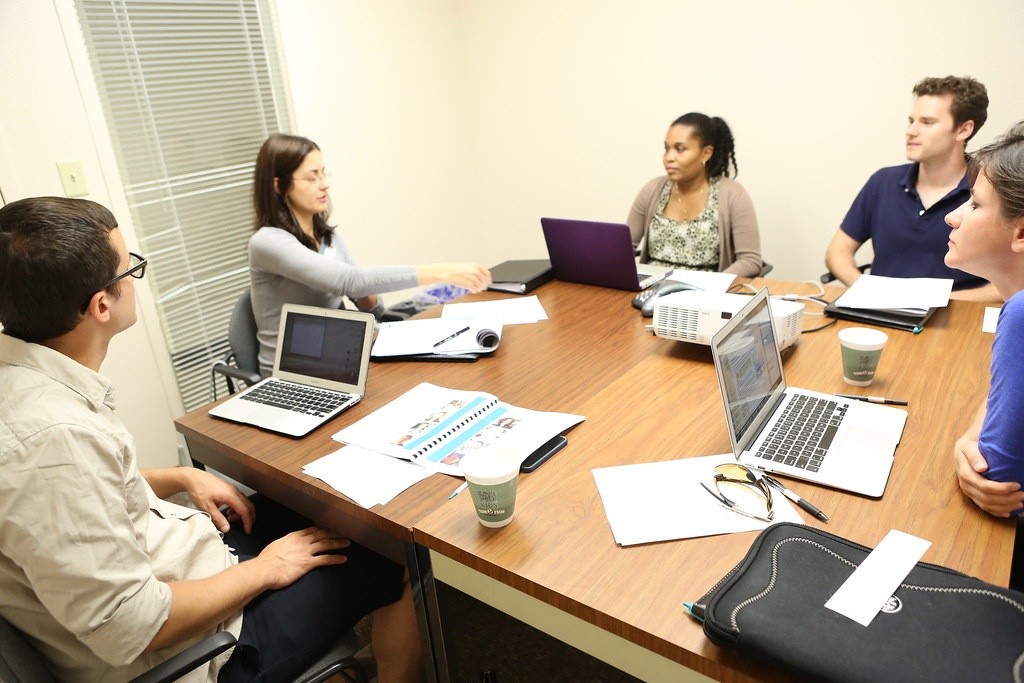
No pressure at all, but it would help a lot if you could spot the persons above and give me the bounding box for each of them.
[943,121,1024,518]
[627,113,762,279]
[390,400,467,448]
[441,415,520,467]
[675,183,705,220]
[248,135,492,382]
[826,76,1007,303]
[0,197,420,683]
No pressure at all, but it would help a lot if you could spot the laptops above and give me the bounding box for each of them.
[207,303,375,438]
[711,288,908,497]
[541,218,673,291]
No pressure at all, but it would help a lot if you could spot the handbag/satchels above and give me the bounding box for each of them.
[703,522,1024,683]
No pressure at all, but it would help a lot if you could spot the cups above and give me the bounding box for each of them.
[838,327,888,387]
[459,447,522,528]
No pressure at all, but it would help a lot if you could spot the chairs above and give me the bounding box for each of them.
[0,614,362,683]
[633,250,870,283]
[213,288,261,388]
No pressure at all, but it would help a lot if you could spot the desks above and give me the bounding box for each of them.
[174,273,1017,683]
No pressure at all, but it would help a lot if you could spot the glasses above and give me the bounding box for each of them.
[81,252,147,314]
[292,172,328,183]
[701,463,774,522]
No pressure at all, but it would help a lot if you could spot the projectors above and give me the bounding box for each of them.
[653,290,804,351]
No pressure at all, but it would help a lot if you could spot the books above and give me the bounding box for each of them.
[331,382,585,477]
[823,287,938,336]
[369,338,480,363]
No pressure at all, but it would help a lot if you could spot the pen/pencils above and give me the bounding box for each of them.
[834,394,908,405]
[450,480,468,499]
[912,326,924,334]
[765,475,830,522]
[433,326,470,347]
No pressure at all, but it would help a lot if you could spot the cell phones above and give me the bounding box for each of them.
[521,435,566,473]
